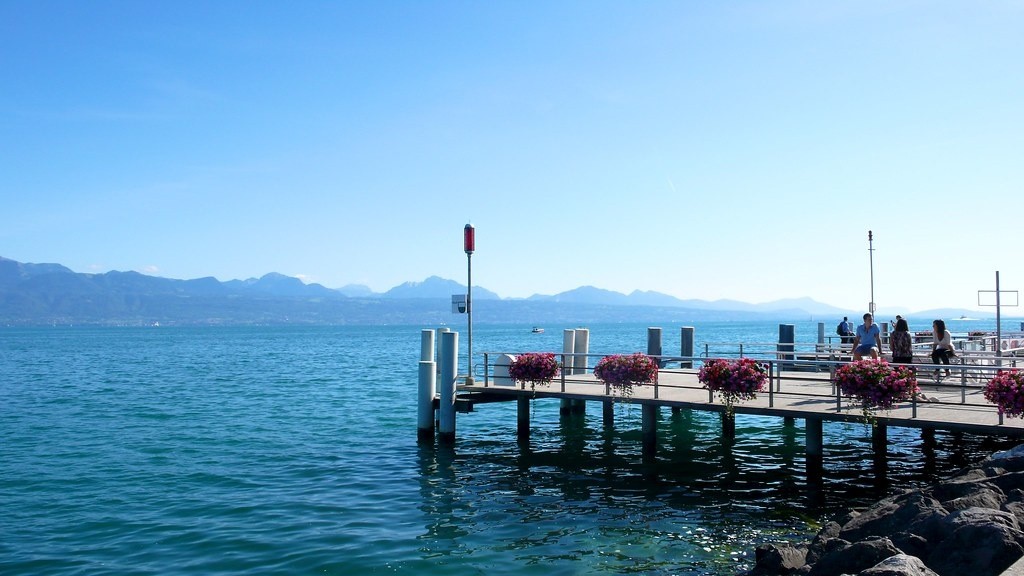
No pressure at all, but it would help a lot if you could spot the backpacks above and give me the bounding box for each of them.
[837,322,844,335]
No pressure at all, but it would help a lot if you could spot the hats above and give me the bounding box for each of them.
[844,317,848,320]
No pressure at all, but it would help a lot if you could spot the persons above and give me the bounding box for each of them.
[840,317,849,353]
[890,315,913,363]
[927,320,957,375]
[847,313,882,360]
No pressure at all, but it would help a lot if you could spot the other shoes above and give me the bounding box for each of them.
[946,372,950,378]
[934,371,939,379]
